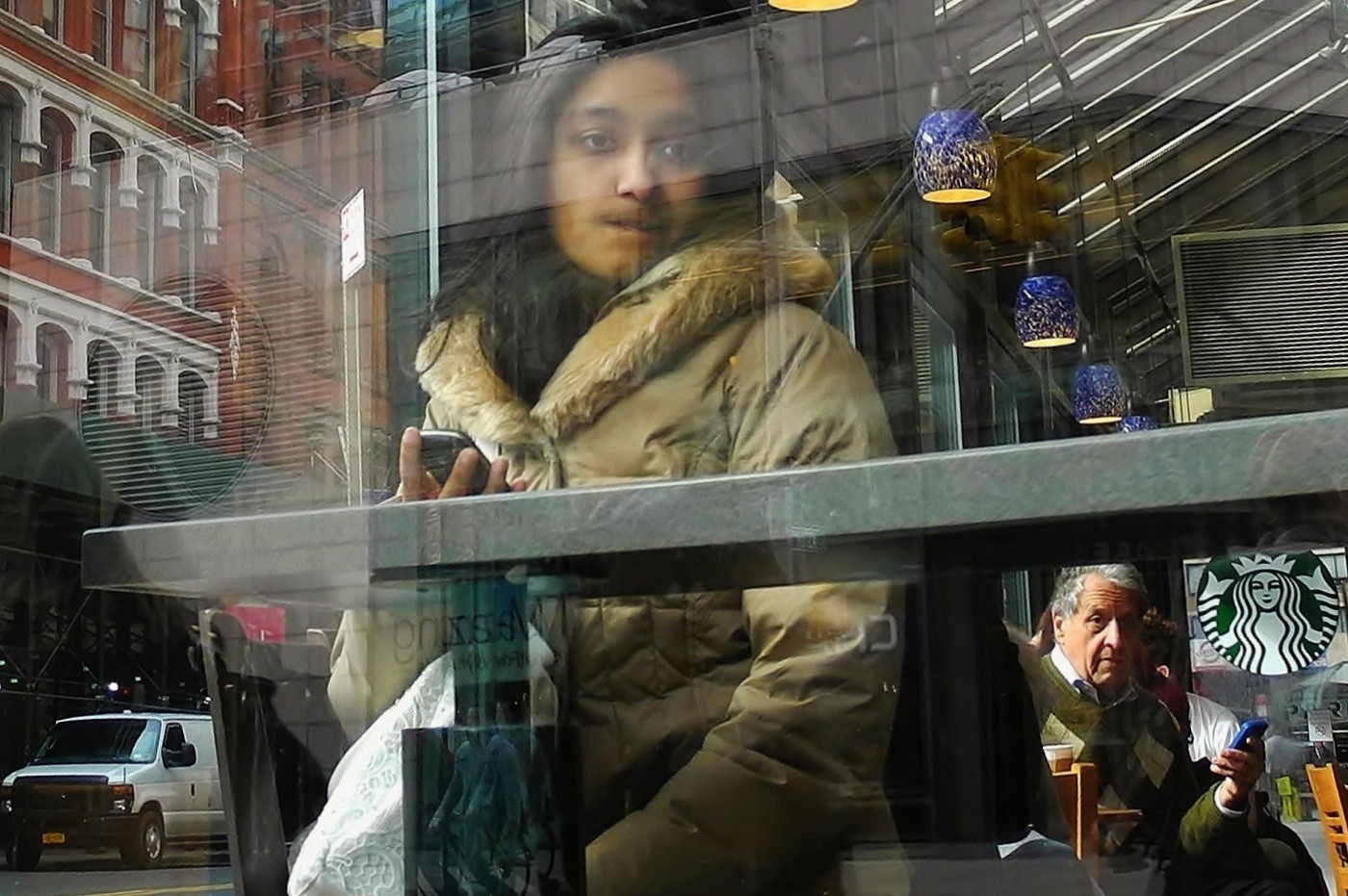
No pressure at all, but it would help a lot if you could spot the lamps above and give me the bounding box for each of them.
[767,0,859,13]
[913,0,998,203]
[1070,97,1128,424]
[1014,1,1080,349]
[1116,200,1158,432]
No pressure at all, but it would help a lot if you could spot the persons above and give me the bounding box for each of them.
[327,6,908,896]
[1006,563,1329,896]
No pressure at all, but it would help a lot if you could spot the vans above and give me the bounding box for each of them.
[0,713,229,873]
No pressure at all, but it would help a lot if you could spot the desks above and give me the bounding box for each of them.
[82,409,1348,896]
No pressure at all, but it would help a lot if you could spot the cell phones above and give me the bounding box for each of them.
[1230,720,1268,751]
[417,429,511,496]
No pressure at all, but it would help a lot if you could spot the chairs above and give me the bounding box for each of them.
[1042,742,1142,885]
[1304,761,1348,896]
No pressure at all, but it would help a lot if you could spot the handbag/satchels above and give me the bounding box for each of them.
[291,616,563,894]
